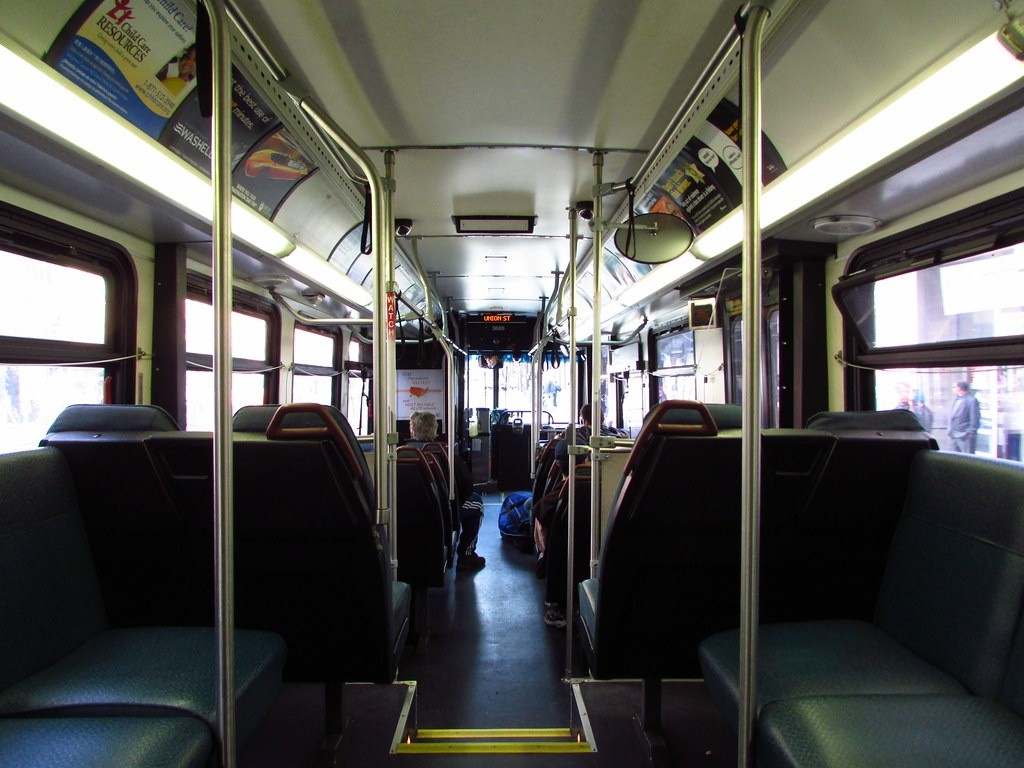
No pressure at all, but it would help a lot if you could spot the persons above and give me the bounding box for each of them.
[531,436,590,629]
[483,354,503,369]
[157,41,198,83]
[946,381,980,454]
[410,411,486,572]
[552,381,561,406]
[581,404,629,437]
[891,393,934,432]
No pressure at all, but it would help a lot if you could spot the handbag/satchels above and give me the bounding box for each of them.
[498,493,534,553]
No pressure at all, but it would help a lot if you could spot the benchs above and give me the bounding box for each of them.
[1,450,274,768]
[699,447,1024,768]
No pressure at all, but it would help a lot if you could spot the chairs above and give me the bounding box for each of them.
[38,405,478,768]
[554,401,940,768]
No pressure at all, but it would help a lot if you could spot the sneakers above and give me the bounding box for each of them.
[544,604,566,628]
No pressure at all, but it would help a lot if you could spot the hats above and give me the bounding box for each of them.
[555,438,589,467]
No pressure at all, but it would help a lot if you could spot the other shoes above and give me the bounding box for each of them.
[456,556,485,572]
